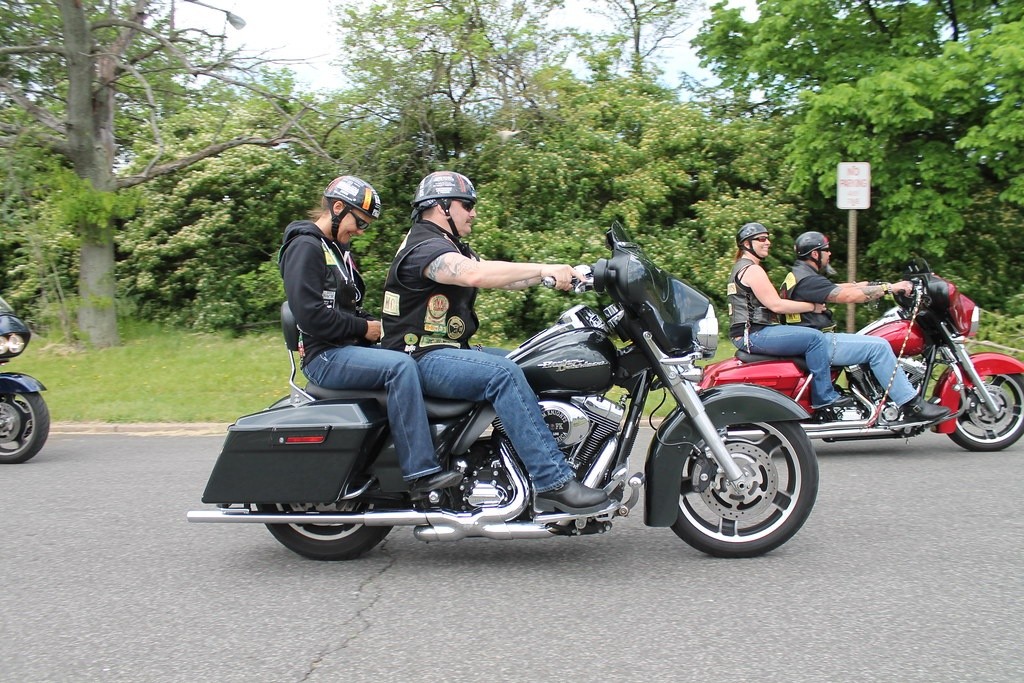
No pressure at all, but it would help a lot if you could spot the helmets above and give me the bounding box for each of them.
[794,231,829,255]
[410,171,477,206]
[324,176,381,219]
[736,223,769,246]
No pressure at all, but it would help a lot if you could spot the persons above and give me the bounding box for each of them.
[380,169,611,514]
[778,230,950,421]
[726,222,853,409]
[277,175,462,500]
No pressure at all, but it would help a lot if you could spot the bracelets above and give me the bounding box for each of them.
[884,285,888,295]
[812,302,816,313]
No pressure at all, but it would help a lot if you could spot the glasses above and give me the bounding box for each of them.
[751,236,770,242]
[452,198,475,212]
[813,248,831,251]
[333,200,369,230]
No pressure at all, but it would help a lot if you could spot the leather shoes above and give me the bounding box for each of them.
[903,396,950,420]
[534,477,611,514]
[827,397,853,406]
[409,470,463,501]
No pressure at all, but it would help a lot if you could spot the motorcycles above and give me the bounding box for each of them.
[697,256,1024,452]
[186,220,820,559]
[0,297,51,463]
